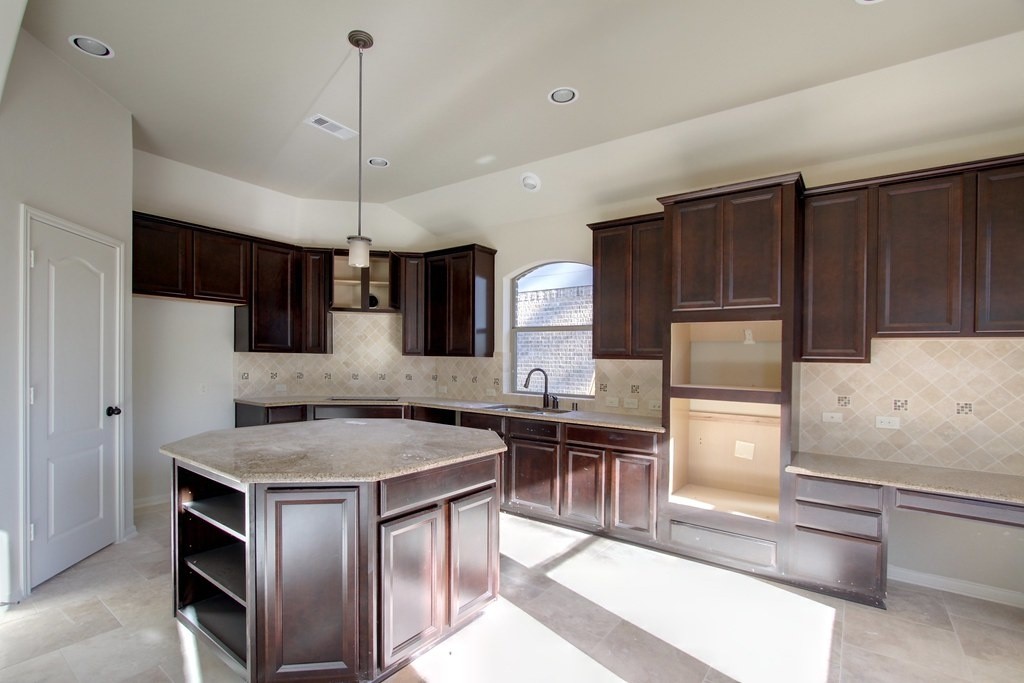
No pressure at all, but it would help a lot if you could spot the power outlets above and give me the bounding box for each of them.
[650,400,661,410]
[624,400,638,408]
[876,416,901,429]
[604,397,618,407]
[824,412,842,423]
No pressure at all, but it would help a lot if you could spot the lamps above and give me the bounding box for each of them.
[346,30,373,267]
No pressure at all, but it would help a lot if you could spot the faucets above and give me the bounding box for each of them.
[524,368,549,408]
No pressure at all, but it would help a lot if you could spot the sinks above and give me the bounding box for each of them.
[484,404,571,417]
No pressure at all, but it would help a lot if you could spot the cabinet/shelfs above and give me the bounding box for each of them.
[656,172,807,321]
[132,211,247,304]
[232,236,326,354]
[396,243,497,358]
[792,478,1024,610]
[661,321,791,588]
[588,212,664,360]
[800,157,1024,363]
[173,404,660,683]
[325,247,401,314]
